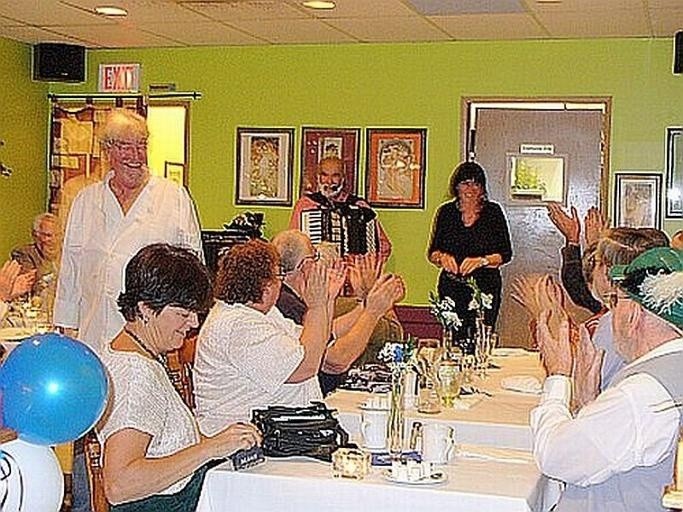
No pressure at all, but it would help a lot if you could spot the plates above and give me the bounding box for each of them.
[0,327,32,341]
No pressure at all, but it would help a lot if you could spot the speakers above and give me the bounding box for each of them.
[31,42,86,83]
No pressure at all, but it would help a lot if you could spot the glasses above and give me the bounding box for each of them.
[297,251,320,269]
[275,268,288,284]
[112,142,149,159]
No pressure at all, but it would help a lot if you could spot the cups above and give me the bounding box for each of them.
[331,340,474,481]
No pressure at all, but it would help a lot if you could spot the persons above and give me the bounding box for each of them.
[508,200,682,512]
[55,155,407,512]
[0,259,38,326]
[54,110,207,512]
[425,162,513,354]
[11,212,61,305]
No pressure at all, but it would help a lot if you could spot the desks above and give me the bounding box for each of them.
[193,445,543,512]
[166,335,199,406]
[325,347,548,449]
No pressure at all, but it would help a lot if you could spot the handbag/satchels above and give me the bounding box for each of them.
[251,401,355,463]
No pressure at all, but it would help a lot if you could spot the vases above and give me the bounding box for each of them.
[474,326,500,380]
[434,362,462,408]
[387,371,429,450]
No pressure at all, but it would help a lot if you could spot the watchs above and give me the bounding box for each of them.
[480,255,489,267]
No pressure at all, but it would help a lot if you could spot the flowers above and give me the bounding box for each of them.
[376,333,416,440]
[428,290,462,353]
[468,276,494,327]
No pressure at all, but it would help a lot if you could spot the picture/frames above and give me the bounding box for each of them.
[164,162,184,184]
[613,172,663,232]
[667,126,683,221]
[299,125,360,198]
[505,153,570,208]
[233,125,295,206]
[363,128,428,210]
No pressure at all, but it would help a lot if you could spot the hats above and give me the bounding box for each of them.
[607,245,683,337]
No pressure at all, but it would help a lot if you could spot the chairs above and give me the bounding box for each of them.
[82,434,111,511]
[0,428,73,512]
